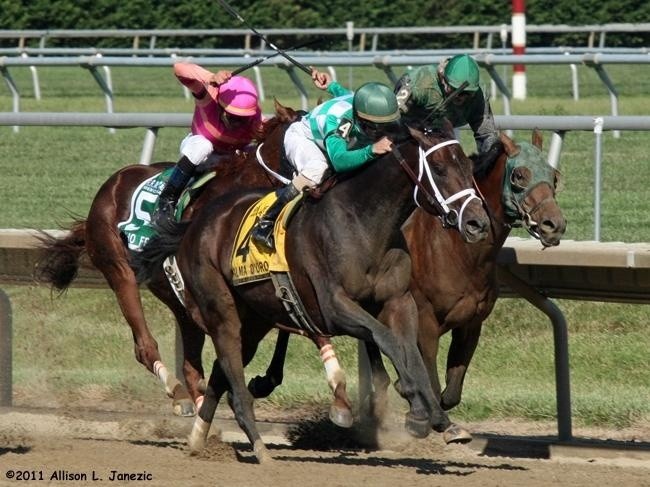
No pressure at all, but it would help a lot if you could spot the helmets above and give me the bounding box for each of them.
[350,80,402,125]
[443,53,481,93]
[216,74,260,120]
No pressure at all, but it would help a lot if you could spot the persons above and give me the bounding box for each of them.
[392,51,501,156]
[250,64,403,250]
[150,60,263,237]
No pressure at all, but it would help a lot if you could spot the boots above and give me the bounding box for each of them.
[152,154,200,236]
[250,182,301,252]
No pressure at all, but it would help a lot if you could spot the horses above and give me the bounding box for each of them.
[132,94,494,471]
[245,124,570,416]
[32,96,357,431]
